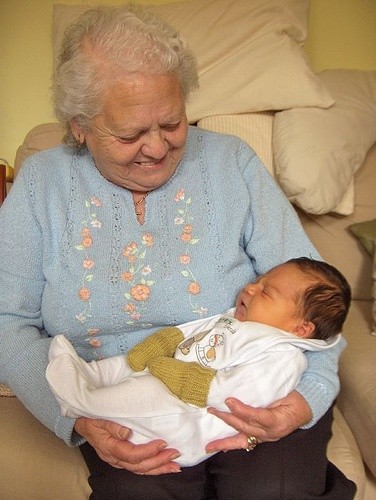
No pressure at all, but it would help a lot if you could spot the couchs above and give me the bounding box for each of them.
[0,71,376,500]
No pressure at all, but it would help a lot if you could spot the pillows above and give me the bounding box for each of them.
[52,1,336,124]
[272,69,376,215]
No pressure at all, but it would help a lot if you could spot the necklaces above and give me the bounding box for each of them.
[133,190,148,216]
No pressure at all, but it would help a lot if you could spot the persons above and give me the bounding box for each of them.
[0,9,358,500]
[44,256,353,468]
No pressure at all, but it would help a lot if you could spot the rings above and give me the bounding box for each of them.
[246,433,258,452]
